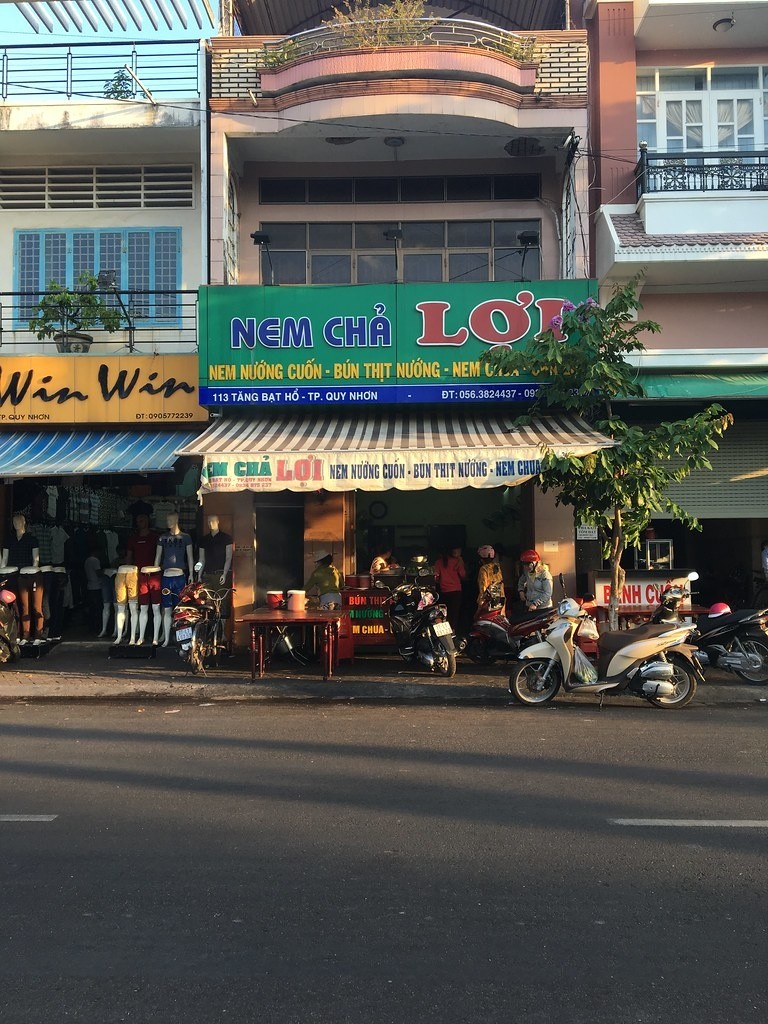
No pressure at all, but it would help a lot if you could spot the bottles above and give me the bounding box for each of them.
[645,528,656,539]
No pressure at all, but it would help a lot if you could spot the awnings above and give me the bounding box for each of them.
[173,412,623,506]
[0,428,206,476]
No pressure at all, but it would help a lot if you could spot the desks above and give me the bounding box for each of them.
[236,608,346,678]
[599,602,712,629]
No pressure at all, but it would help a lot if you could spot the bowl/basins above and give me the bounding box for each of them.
[346,575,371,590]
[373,574,403,588]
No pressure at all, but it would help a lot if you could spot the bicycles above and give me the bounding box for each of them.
[186,584,237,676]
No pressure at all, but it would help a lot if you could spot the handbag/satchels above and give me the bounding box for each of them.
[573,647,599,684]
[483,583,505,611]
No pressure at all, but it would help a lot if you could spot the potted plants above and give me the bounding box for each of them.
[28,278,118,352]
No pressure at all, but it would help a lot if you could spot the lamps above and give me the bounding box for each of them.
[249,231,281,288]
[713,18,735,34]
[384,229,406,285]
[518,230,539,282]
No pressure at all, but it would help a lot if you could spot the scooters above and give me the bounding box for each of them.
[0,579,22,665]
[374,566,458,678]
[509,572,707,709]
[462,564,565,667]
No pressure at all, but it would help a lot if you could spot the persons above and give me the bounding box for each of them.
[154,512,194,648]
[477,544,506,617]
[638,530,675,569]
[302,550,345,612]
[370,544,398,575]
[197,513,233,642]
[110,543,133,568]
[135,566,162,645]
[98,568,118,638]
[434,543,467,637]
[84,544,109,623]
[761,541,768,580]
[122,514,159,637]
[517,550,553,611]
[112,565,139,644]
[0,515,69,646]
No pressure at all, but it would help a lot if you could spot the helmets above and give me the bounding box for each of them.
[520,550,541,561]
[708,602,732,619]
[477,545,495,558]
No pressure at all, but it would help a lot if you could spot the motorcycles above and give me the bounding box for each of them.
[161,560,211,671]
[638,572,768,686]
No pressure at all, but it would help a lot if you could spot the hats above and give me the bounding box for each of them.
[313,549,328,563]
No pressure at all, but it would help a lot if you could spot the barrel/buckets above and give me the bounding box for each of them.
[267,591,283,610]
[286,589,306,611]
